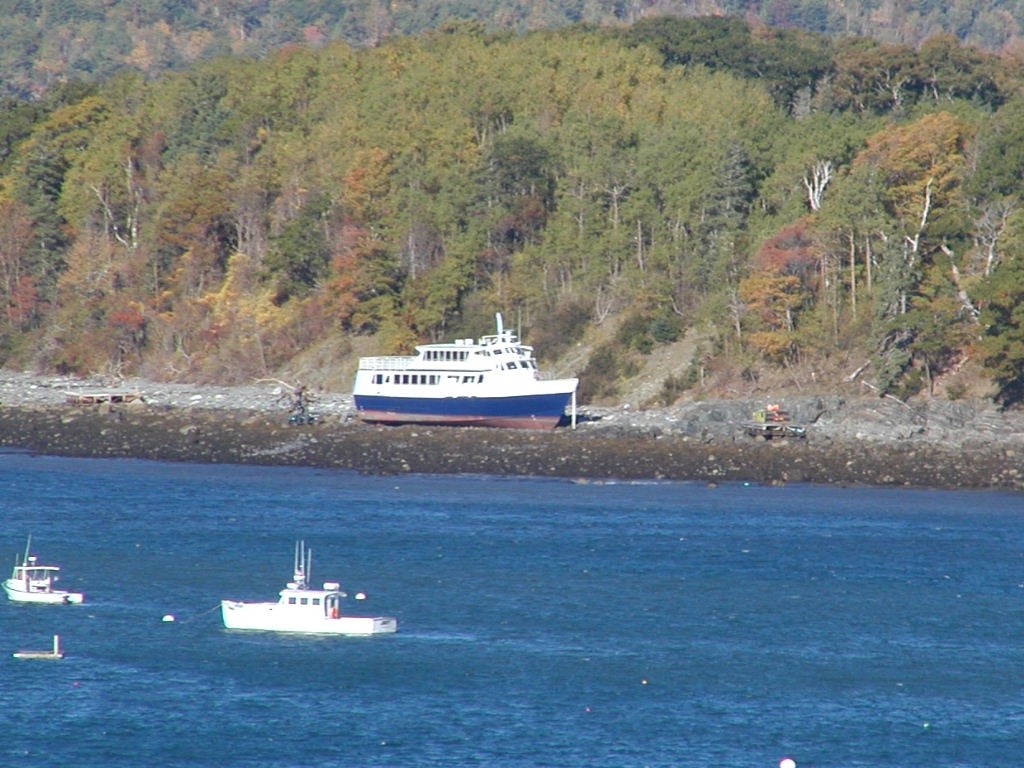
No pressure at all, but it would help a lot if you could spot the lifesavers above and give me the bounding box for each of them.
[332,608,341,619]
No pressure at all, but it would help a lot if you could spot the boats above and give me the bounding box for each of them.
[349,312,580,432]
[11,633,64,662]
[1,532,84,606]
[221,538,397,635]
